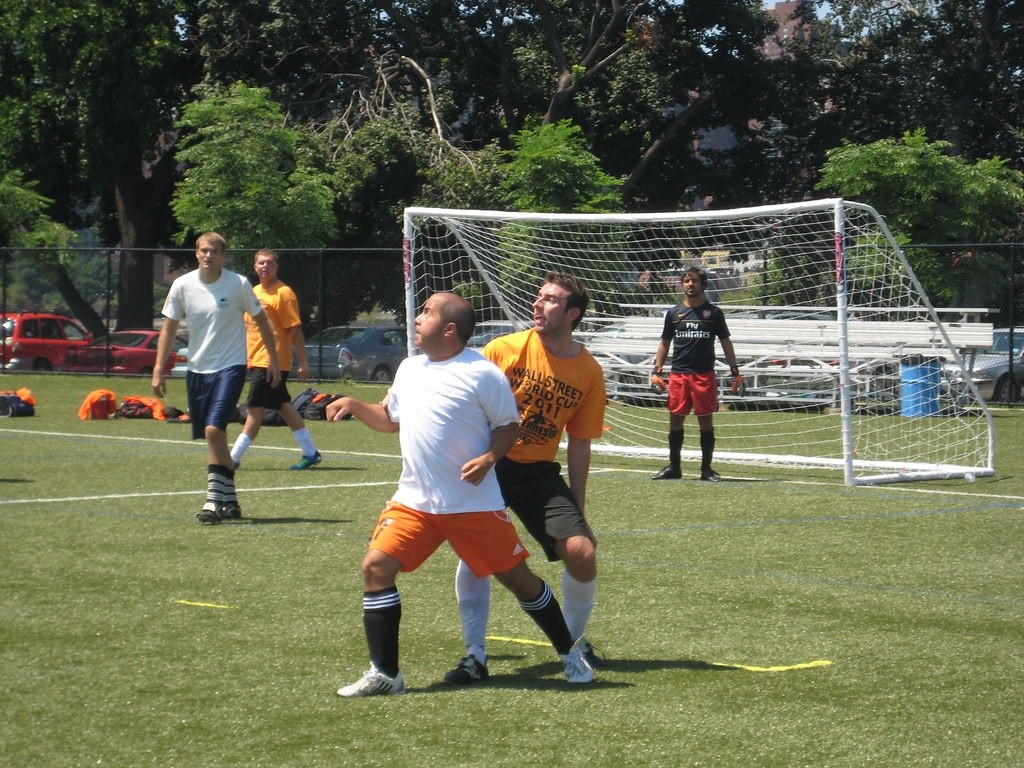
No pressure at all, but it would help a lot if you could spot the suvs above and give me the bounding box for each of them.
[0,311,96,373]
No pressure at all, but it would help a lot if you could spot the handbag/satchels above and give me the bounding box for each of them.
[0,394,36,416]
[291,388,353,421]
[238,403,287,426]
[120,396,153,419]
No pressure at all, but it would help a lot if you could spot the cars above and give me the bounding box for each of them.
[938,325,1024,408]
[304,322,408,385]
[60,329,190,373]
[169,344,300,379]
[464,318,618,400]
[594,316,899,418]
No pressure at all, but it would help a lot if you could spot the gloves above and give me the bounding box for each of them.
[651,366,666,396]
[731,367,746,397]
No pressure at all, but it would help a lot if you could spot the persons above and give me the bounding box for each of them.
[651,267,746,482]
[444,274,607,687]
[230,248,322,470]
[152,232,281,524]
[326,292,593,698]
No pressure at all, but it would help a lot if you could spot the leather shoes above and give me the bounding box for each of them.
[701,469,722,482]
[651,465,681,478]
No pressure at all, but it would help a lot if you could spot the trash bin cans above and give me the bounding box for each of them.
[899,356,944,417]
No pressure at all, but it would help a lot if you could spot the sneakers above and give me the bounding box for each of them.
[290,449,322,470]
[196,502,223,525]
[558,634,594,683]
[445,654,487,685]
[221,500,241,517]
[578,641,603,668]
[337,661,403,698]
